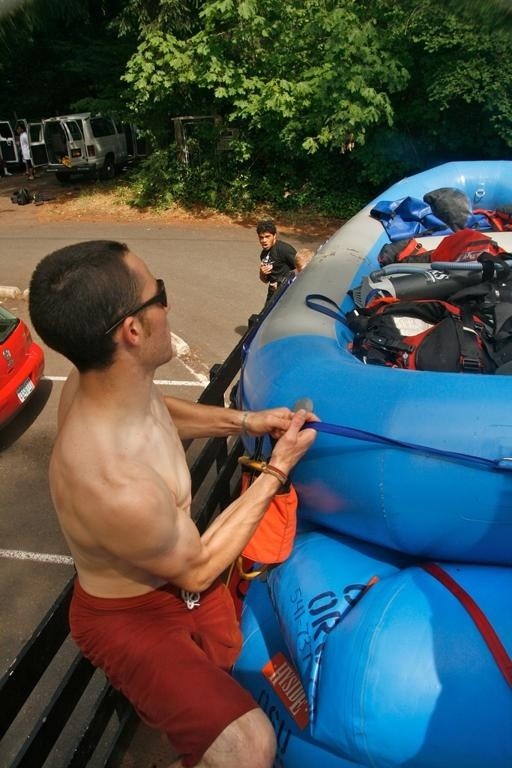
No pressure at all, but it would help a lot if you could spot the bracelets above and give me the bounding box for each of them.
[261,462,288,486]
[242,409,250,438]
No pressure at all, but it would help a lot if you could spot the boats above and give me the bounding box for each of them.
[233,156,512,569]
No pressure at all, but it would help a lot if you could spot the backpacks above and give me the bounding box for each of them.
[344,228,512,374]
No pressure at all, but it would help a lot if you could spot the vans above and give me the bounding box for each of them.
[26,109,138,184]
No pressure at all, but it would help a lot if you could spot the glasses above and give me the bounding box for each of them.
[101,279,167,341]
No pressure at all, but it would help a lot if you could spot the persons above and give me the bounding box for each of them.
[14,125,35,181]
[0,145,13,178]
[254,220,307,300]
[27,238,320,768]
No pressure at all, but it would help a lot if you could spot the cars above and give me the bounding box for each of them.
[0,305,46,433]
